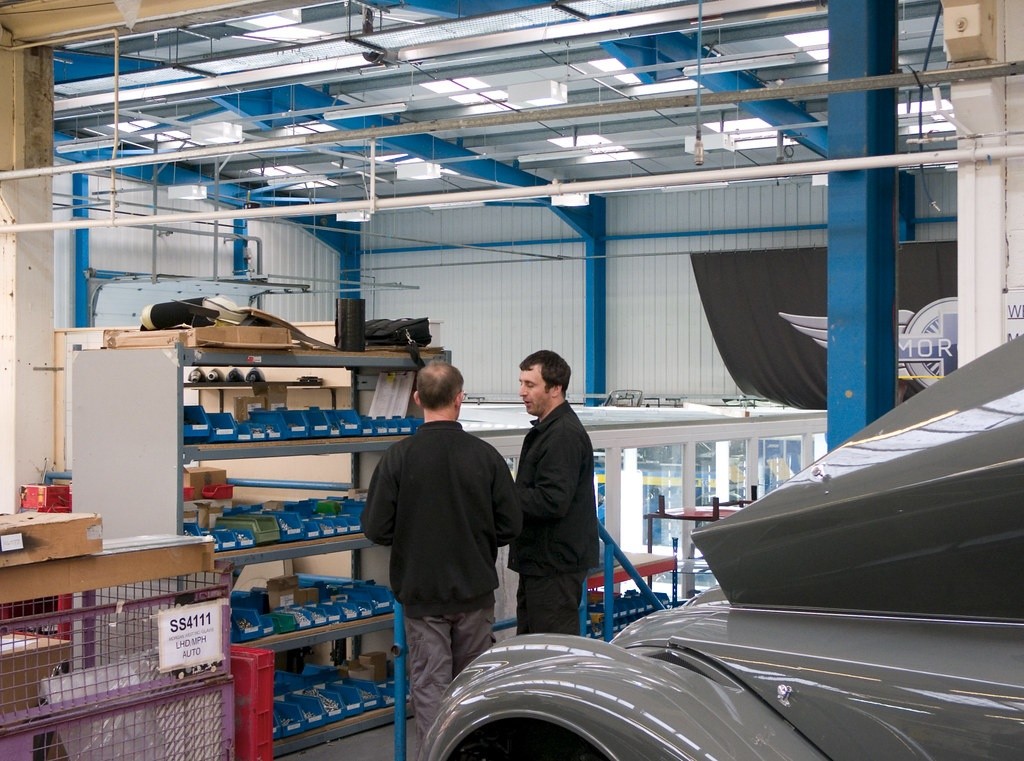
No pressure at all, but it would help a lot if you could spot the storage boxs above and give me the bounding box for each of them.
[229,643,275,761]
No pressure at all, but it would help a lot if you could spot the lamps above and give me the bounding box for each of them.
[103,5,957,222]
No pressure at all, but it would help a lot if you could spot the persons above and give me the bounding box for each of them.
[505,350,601,642]
[362,363,520,754]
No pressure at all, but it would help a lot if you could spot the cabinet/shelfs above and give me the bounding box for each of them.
[72,347,831,759]
[0,532,235,761]
